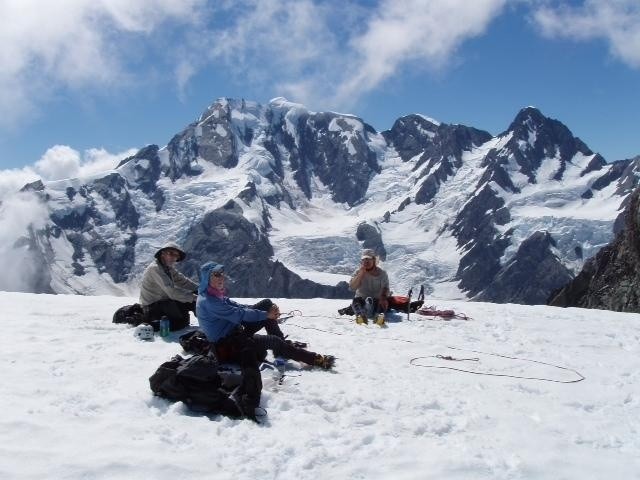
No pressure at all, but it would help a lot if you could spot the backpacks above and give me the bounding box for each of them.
[150,354,262,419]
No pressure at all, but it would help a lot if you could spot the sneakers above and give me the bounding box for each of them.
[315,354,334,370]
[375,312,384,324]
[356,313,367,324]
[283,338,306,347]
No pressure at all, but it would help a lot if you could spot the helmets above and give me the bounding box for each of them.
[134,323,153,339]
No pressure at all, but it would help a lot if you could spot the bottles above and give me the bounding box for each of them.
[159,316,169,337]
[274,358,285,375]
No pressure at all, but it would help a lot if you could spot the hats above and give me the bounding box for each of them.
[154,242,185,261]
[361,248,375,260]
[198,261,224,296]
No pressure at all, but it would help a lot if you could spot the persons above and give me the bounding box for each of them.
[195,260,335,370]
[349,248,390,326]
[138,242,199,332]
